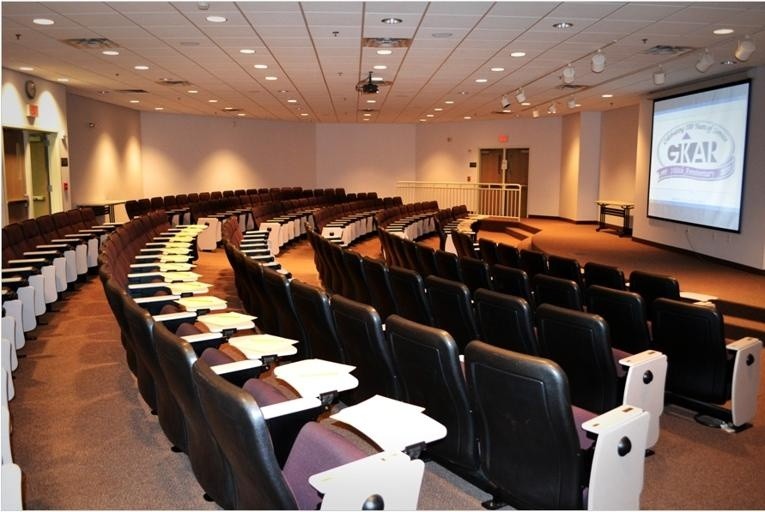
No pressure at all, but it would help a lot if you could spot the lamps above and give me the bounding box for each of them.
[591,55,605,73]
[516,94,526,104]
[563,68,575,85]
[500,98,510,108]
[734,41,756,61]
[545,104,556,114]
[532,109,538,119]
[696,54,714,73]
[654,71,664,85]
[567,97,576,109]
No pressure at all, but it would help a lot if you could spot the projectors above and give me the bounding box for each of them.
[363,72,379,94]
